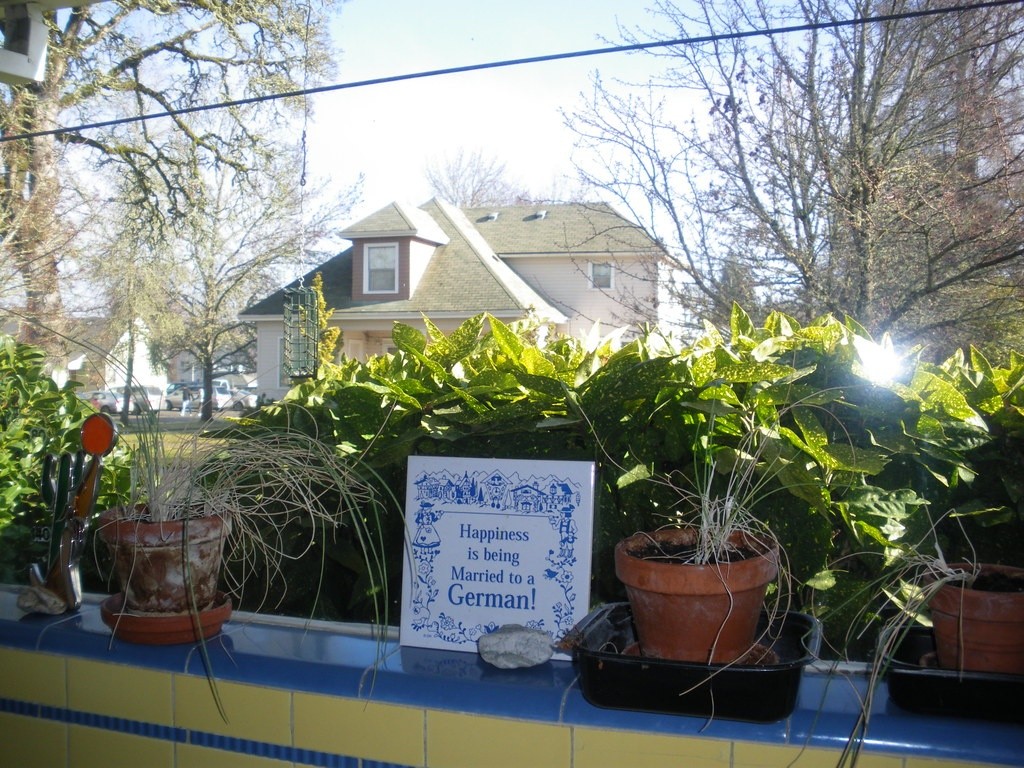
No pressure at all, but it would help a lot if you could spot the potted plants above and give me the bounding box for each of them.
[18,310,411,716]
[798,497,1024,762]
[562,367,853,664]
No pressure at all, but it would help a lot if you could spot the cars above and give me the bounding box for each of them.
[81,379,255,416]
